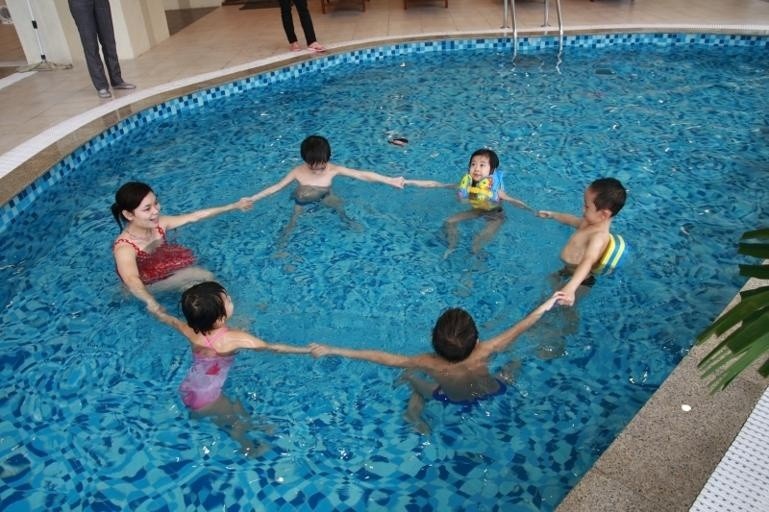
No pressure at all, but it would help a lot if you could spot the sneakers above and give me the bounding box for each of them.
[114,82,135,88]
[98,88,112,98]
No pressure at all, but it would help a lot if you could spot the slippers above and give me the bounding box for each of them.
[290,44,301,50]
[307,42,325,52]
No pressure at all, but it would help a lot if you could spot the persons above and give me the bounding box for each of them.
[311,291,566,437]
[241,134,404,270]
[536,177,627,307]
[147,278,311,457]
[279,0,327,53]
[404,148,537,254]
[110,182,250,312]
[68,0,137,99]
